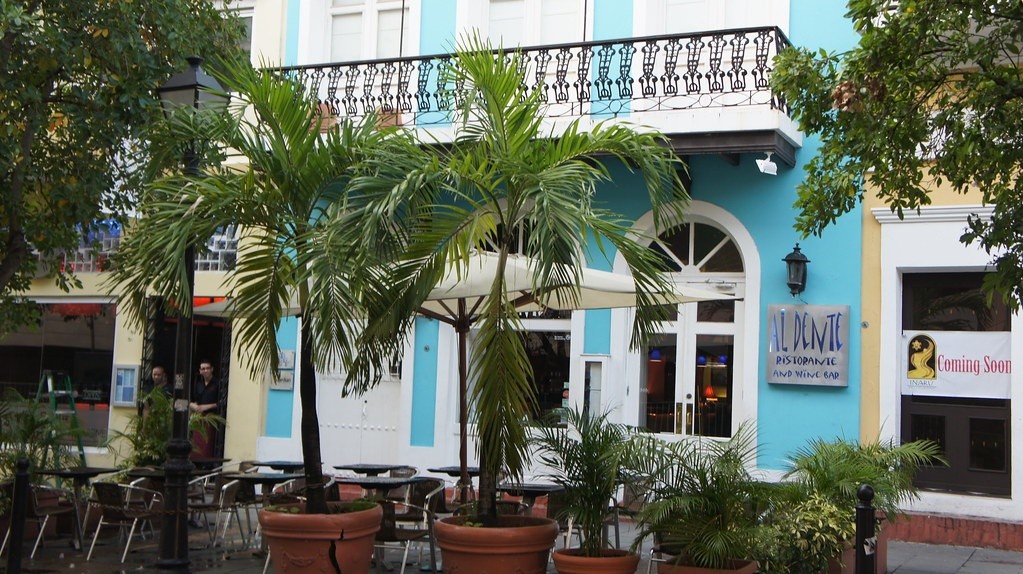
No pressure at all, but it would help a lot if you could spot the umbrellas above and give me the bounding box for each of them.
[194,246,744,516]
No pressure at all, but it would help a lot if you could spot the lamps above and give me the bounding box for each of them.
[755,151,780,177]
[782,243,812,298]
[702,384,716,398]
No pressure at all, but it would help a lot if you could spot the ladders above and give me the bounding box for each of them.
[20,370,90,490]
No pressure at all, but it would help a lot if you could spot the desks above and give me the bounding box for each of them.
[564,472,652,549]
[126,468,219,481]
[335,464,409,501]
[223,473,305,559]
[332,478,421,567]
[496,483,565,515]
[428,467,503,477]
[251,461,323,474]
[36,466,120,551]
[189,457,232,466]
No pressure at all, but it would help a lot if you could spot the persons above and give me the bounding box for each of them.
[189,360,220,459]
[142,366,171,422]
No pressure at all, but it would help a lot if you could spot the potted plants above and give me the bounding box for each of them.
[107,46,444,574]
[347,48,691,574]
[784,438,951,574]
[534,403,643,574]
[631,431,778,574]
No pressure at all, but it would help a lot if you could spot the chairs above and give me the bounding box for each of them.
[0,461,774,574]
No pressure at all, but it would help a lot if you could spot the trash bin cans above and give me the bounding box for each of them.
[623,472,653,511]
